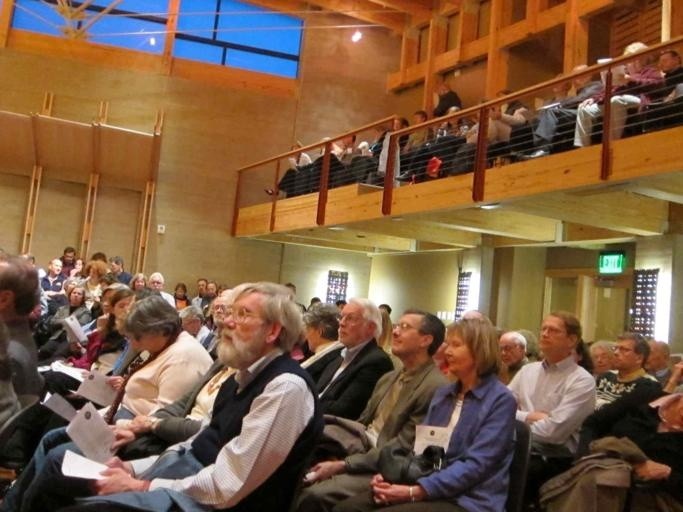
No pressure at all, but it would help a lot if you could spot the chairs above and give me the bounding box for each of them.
[0,393,42,455]
[507,418,536,511]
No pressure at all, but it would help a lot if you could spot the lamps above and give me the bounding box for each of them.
[123,14,391,46]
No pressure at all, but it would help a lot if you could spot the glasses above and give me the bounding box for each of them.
[392,323,419,331]
[224,308,261,324]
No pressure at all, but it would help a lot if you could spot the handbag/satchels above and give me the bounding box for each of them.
[375,439,447,486]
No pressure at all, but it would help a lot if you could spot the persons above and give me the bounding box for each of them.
[263,43,683,195]
[1,247,683,512]
